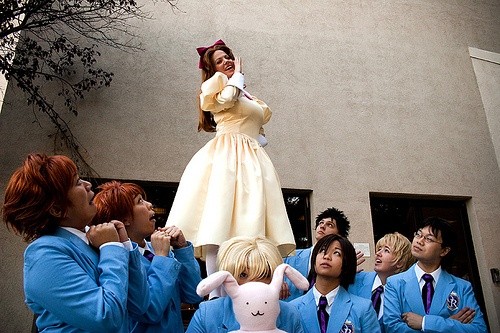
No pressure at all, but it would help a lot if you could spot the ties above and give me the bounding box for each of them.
[143,249,155,262]
[420,273,435,314]
[371,285,384,318]
[303,273,316,294]
[317,296,330,333]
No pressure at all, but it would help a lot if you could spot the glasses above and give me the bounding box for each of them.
[414,231,442,245]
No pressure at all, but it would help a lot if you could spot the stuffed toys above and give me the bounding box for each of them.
[195,263,309,333]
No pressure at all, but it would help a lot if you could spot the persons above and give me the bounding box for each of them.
[382,217,488,333]
[348,232,416,321]
[165,41,297,299]
[91,181,204,333]
[289,234,381,333]
[185,235,304,333]
[0,154,151,333]
[279,207,350,302]
